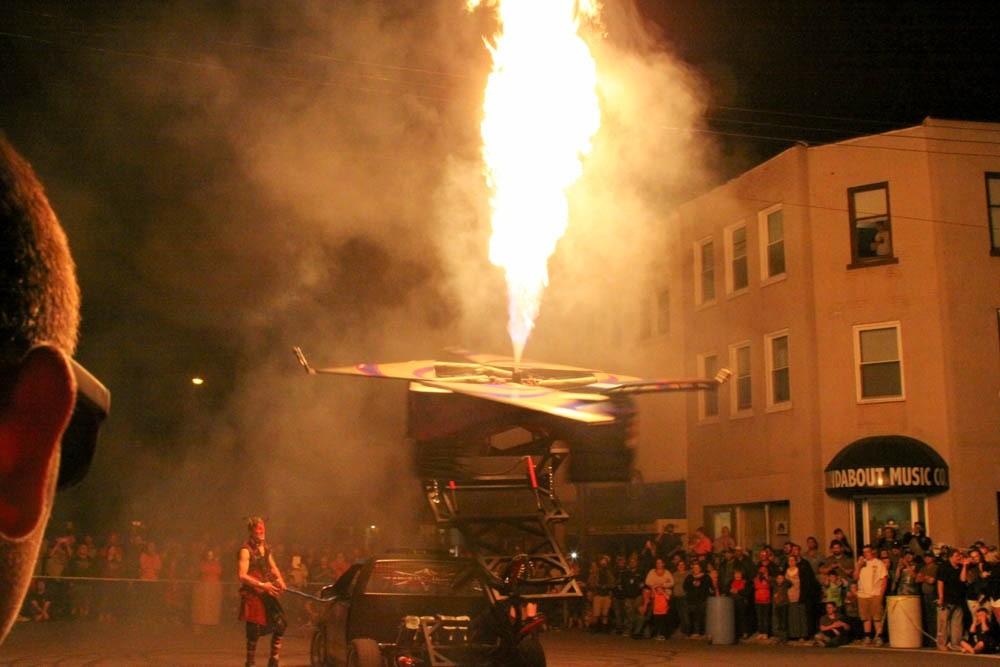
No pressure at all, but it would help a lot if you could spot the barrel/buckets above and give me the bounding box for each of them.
[704,597,735,645]
[885,595,922,649]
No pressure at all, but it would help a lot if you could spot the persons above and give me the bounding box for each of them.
[238,516,286,667]
[0,133,110,650]
[554,519,1000,656]
[19,521,365,636]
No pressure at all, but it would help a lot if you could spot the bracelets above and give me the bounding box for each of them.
[256,581,265,590]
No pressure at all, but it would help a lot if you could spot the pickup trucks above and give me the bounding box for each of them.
[311,546,547,667]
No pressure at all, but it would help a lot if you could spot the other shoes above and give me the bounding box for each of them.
[862,637,872,648]
[655,635,666,640]
[873,637,884,648]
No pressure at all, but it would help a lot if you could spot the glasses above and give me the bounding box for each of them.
[28,340,114,493]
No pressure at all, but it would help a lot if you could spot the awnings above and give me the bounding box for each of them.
[824,433,949,501]
